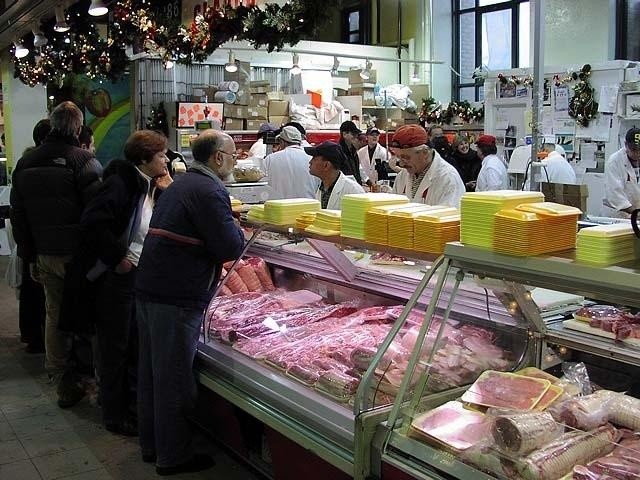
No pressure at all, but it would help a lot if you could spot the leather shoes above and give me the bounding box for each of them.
[106,416,140,436]
[157,454,214,474]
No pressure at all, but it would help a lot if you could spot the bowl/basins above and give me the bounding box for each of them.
[229,171,264,182]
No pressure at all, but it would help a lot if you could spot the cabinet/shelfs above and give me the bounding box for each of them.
[379,243,640,480]
[200,222,447,480]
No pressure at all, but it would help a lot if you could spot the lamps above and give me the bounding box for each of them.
[225,51,238,73]
[32,22,49,47]
[86,0,109,17]
[53,6,71,32]
[330,56,340,76]
[289,55,302,75]
[12,40,29,59]
[360,62,372,79]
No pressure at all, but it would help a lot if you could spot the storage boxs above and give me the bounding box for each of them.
[348,88,375,106]
[268,117,290,128]
[349,69,377,85]
[266,91,285,101]
[408,85,429,112]
[246,107,267,119]
[250,87,270,93]
[191,83,217,102]
[268,102,289,116]
[248,95,269,107]
[224,62,250,106]
[376,109,417,120]
[226,118,244,130]
[245,120,268,129]
[223,102,248,120]
[374,119,419,130]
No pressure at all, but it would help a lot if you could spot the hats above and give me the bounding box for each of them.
[259,124,278,132]
[340,121,362,133]
[474,135,496,145]
[304,141,347,168]
[540,134,556,143]
[366,127,380,134]
[392,125,436,148]
[281,123,305,134]
[626,127,640,147]
[277,126,301,144]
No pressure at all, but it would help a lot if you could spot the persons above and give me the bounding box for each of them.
[272,122,313,154]
[390,126,467,210]
[451,133,482,191]
[248,123,275,162]
[473,135,510,190]
[77,126,97,158]
[603,128,640,218]
[336,122,363,185]
[260,126,321,199]
[11,101,105,378]
[427,123,465,185]
[305,142,366,211]
[356,128,391,184]
[131,130,244,476]
[78,134,178,433]
[18,119,55,352]
[523,135,577,193]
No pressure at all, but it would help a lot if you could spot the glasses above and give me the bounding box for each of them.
[218,150,238,162]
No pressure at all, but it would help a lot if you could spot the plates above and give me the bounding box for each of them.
[535,384,564,413]
[200,321,231,333]
[265,197,341,236]
[239,205,264,223]
[315,382,349,402]
[342,192,460,254]
[460,190,583,258]
[346,400,355,408]
[285,368,317,387]
[461,370,552,413]
[235,331,251,340]
[514,365,581,397]
[231,340,266,360]
[559,452,612,480]
[264,332,319,371]
[576,222,640,266]
[410,400,489,453]
